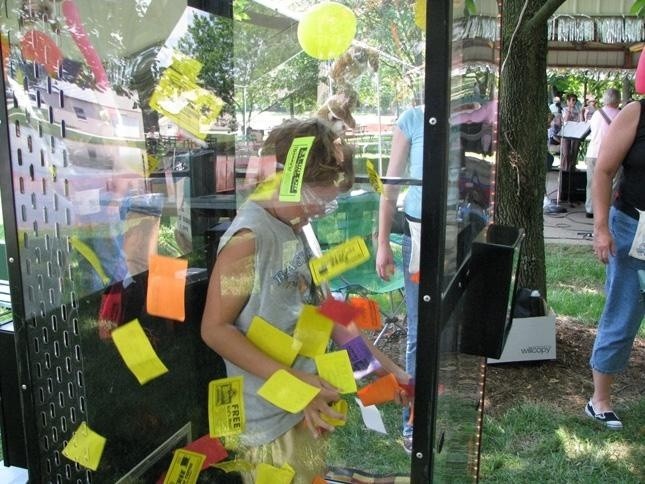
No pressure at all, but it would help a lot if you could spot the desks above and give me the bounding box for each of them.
[128,186,381,331]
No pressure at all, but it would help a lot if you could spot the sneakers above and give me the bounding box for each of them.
[585,213,594,219]
[583,395,624,431]
[404,438,412,452]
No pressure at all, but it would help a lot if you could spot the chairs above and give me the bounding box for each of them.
[316,191,412,350]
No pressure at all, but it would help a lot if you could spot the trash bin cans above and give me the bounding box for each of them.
[474,221,527,360]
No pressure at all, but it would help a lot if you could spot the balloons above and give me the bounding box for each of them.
[415,0,429,32]
[296,3,359,63]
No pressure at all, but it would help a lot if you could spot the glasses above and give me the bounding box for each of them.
[273,160,339,221]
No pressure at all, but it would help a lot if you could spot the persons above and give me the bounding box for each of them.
[583,96,600,124]
[583,86,622,219]
[65,92,135,299]
[583,92,644,434]
[558,93,588,177]
[371,102,424,457]
[197,115,414,484]
[547,95,564,156]
